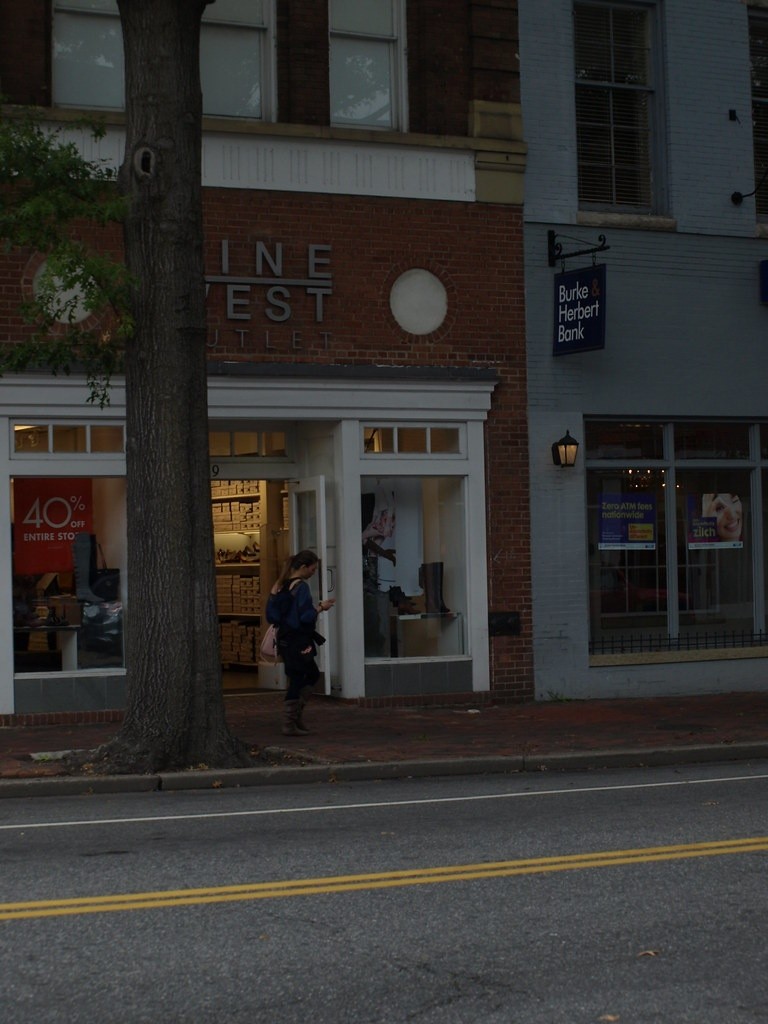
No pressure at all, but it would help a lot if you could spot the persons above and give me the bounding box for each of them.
[702,494,742,542]
[362,480,396,567]
[275,550,336,736]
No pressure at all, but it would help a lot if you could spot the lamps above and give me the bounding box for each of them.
[551,430,579,467]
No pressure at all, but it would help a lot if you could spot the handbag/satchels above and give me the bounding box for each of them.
[259,623,278,666]
[93,544,119,600]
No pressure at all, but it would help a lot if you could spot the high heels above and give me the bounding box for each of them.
[389,586,421,615]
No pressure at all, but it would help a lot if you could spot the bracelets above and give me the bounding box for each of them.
[319,602,323,611]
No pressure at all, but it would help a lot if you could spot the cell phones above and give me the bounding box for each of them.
[328,599,337,603]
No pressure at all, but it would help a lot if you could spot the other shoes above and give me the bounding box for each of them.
[217,541,260,561]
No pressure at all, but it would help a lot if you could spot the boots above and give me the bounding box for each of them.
[365,539,396,567]
[422,563,441,613]
[286,699,310,736]
[432,562,450,613]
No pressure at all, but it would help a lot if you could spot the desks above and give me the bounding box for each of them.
[13,625,81,671]
[389,612,467,657]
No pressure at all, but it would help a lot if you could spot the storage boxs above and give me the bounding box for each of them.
[48,594,82,625]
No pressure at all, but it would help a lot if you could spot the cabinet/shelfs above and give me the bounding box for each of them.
[211,480,289,669]
